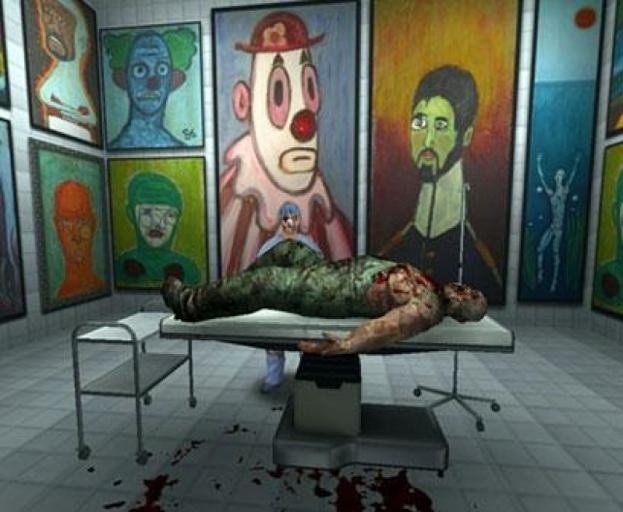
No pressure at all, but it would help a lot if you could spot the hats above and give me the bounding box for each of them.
[235,12,325,53]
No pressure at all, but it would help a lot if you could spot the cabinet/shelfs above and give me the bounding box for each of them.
[72,296,196,467]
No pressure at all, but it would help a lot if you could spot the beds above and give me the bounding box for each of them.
[160,306,513,473]
